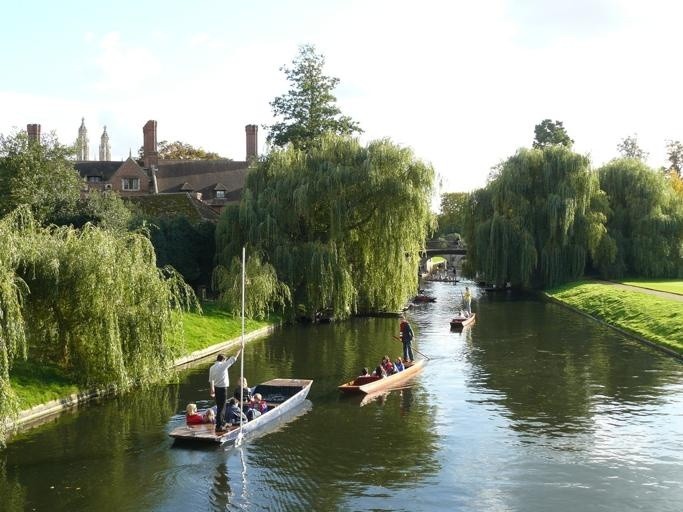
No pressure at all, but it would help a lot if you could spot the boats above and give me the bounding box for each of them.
[449,318,475,334]
[426,275,459,282]
[335,377,423,407]
[170,376,313,444]
[449,312,476,328]
[339,357,427,396]
[484,285,511,292]
[415,292,437,302]
[170,399,314,451]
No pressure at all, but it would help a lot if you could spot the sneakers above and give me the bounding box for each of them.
[214,422,233,433]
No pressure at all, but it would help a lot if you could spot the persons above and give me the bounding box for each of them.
[249,393,269,413]
[486,279,512,288]
[453,267,456,280]
[202,409,216,424]
[375,365,386,378]
[208,343,243,435]
[457,237,460,249]
[457,309,470,318]
[245,407,261,420]
[419,288,425,296]
[358,368,370,377]
[382,355,398,375]
[225,398,247,424]
[464,287,471,314]
[186,403,203,424]
[418,271,422,277]
[426,271,452,281]
[399,315,415,362]
[395,357,405,371]
[377,387,415,420]
[233,377,251,401]
